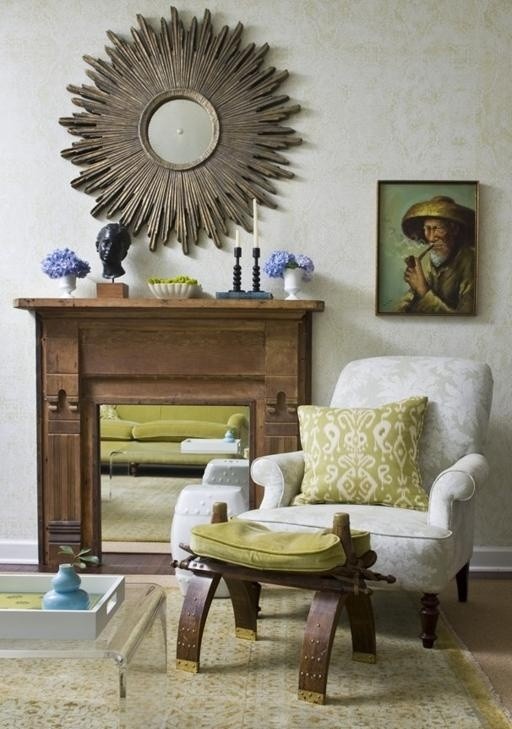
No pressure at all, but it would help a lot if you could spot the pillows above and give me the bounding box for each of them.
[293,394,434,511]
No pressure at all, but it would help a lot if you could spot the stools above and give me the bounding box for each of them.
[170,482,255,605]
[169,500,398,704]
[200,458,249,508]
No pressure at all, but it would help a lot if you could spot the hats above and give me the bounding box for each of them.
[401,195,475,245]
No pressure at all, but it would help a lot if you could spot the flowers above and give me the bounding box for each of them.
[262,248,318,278]
[40,245,91,276]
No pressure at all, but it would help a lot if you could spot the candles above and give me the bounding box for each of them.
[253,197,259,247]
[235,227,241,249]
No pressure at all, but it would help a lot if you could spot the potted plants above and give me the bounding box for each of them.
[147,274,200,303]
[35,542,102,613]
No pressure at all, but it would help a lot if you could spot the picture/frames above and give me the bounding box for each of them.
[373,176,484,320]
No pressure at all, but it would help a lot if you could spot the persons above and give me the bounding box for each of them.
[399,196,476,315]
[95,223,131,278]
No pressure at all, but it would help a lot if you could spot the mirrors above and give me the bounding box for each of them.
[97,404,255,559]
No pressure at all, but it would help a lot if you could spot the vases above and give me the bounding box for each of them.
[61,274,79,301]
[281,267,304,303]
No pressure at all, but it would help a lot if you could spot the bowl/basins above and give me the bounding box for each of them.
[148,281,202,299]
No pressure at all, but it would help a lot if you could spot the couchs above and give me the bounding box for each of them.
[99,402,250,476]
[249,352,497,651]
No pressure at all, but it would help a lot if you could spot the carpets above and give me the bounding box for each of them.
[1,566,512,729]
